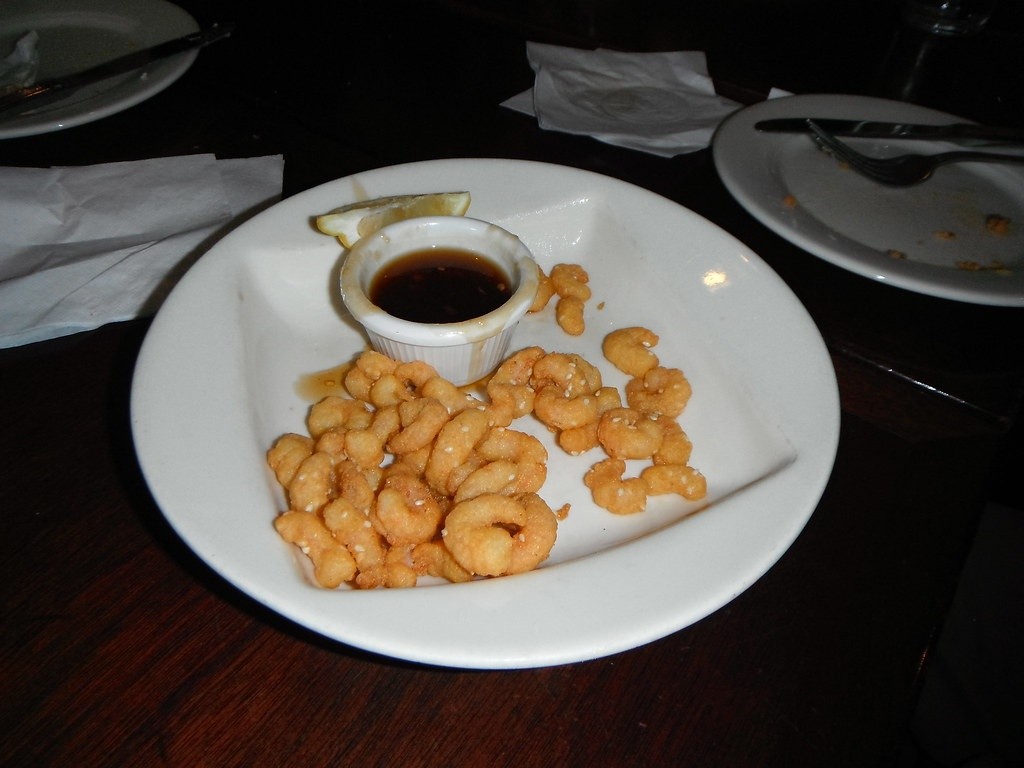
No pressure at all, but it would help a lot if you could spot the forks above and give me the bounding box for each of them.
[806,118,1024,188]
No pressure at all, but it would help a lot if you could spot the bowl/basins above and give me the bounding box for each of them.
[338,214,540,386]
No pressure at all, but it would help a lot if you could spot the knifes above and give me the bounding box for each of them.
[754,116,1024,148]
[0,23,231,125]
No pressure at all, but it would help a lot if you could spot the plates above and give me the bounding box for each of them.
[130,157,841,670]
[712,93,1024,308]
[0,0,202,140]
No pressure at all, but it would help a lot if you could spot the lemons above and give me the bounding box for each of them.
[316,191,472,249]
